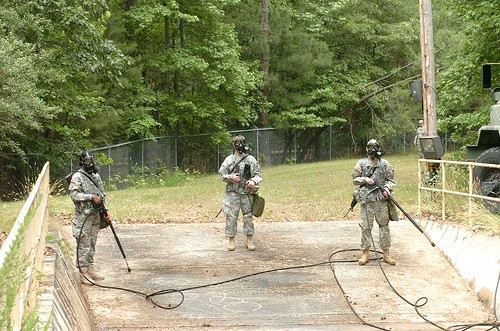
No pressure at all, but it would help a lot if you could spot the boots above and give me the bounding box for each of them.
[78,267,96,286]
[358,250,370,264]
[383,249,395,265]
[226,235,238,251]
[246,236,256,250]
[87,263,105,280]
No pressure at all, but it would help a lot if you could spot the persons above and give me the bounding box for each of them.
[414,120,424,159]
[219,135,263,251]
[69,150,112,285]
[351,139,396,264]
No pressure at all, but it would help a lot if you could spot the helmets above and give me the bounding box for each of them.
[233,135,247,153]
[366,139,381,156]
[79,149,95,170]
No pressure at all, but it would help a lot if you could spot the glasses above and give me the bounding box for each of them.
[367,145,380,152]
[234,141,245,147]
[84,158,95,165]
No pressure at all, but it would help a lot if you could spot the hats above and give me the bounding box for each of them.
[419,120,423,124]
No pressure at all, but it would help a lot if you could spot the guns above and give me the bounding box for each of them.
[343,199,357,220]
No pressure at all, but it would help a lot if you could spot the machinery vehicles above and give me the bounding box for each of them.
[461,62,500,217]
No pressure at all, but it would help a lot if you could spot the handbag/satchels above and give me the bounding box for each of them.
[387,201,398,221]
[99,213,110,230]
[249,194,265,217]
